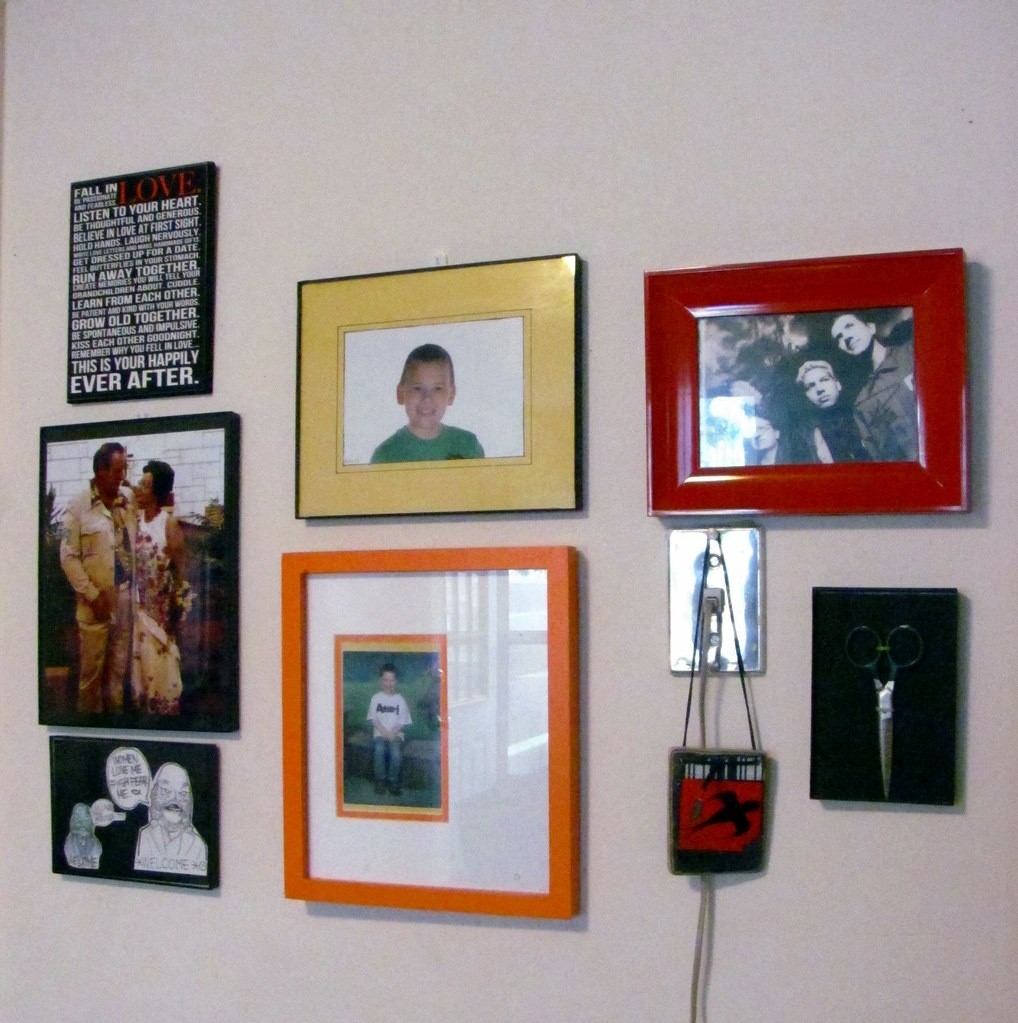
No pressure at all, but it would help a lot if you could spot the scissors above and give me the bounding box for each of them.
[841,626,925,801]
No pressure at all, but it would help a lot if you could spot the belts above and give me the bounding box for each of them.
[115,580,130,592]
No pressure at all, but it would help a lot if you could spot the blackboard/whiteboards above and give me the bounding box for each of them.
[66,160,220,405]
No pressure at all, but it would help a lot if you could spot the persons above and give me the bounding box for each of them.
[367,663,412,797]
[59,441,197,717]
[371,344,484,464]
[745,415,781,466]
[774,359,875,464]
[828,313,918,462]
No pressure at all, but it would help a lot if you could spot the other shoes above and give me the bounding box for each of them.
[375,779,386,794]
[387,780,401,795]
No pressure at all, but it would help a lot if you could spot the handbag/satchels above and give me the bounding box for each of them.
[666,748,766,876]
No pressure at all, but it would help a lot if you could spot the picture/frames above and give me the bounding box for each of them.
[295,250,587,519]
[641,247,970,519]
[46,736,221,893]
[37,410,243,737]
[282,546,581,919]
